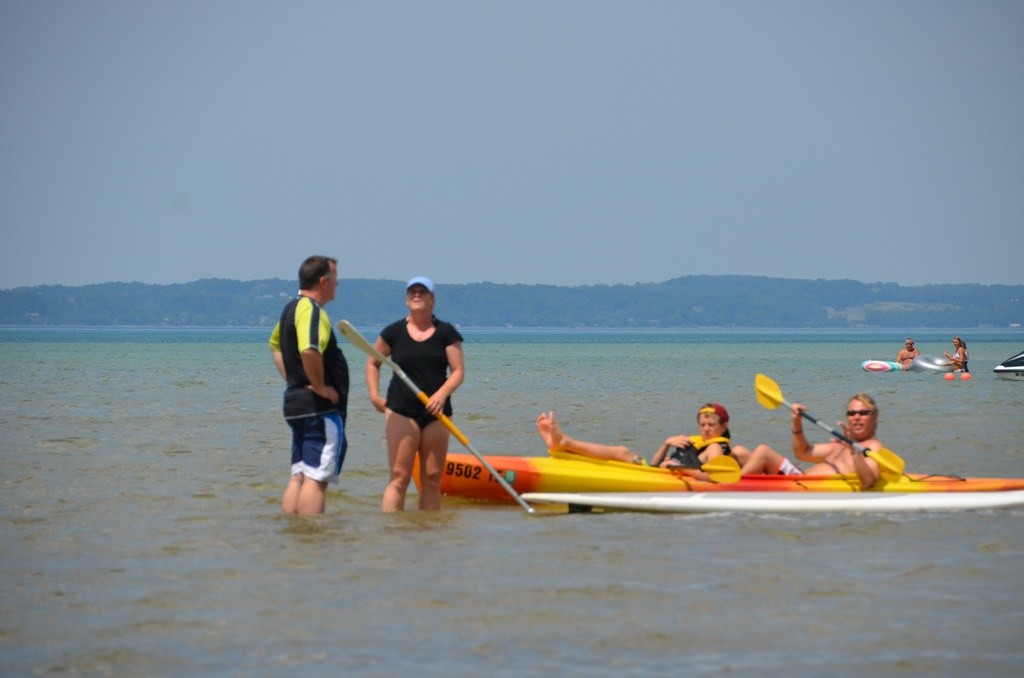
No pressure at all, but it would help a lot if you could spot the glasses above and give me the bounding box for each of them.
[846,410,875,416]
[905,343,914,345]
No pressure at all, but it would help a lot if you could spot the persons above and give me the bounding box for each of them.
[729,392,884,489]
[944,336,964,373]
[268,255,350,514]
[365,275,465,513]
[896,337,920,370]
[961,342,970,372]
[536,403,736,483]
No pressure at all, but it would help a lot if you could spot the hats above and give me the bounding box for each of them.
[697,403,729,423]
[407,276,435,295]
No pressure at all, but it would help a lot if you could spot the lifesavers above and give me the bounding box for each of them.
[913,352,954,374]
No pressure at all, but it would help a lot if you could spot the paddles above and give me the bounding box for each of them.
[336,318,535,518]
[754,371,907,481]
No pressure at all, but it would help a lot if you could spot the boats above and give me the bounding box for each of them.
[409,451,1024,507]
[993,351,1024,384]
